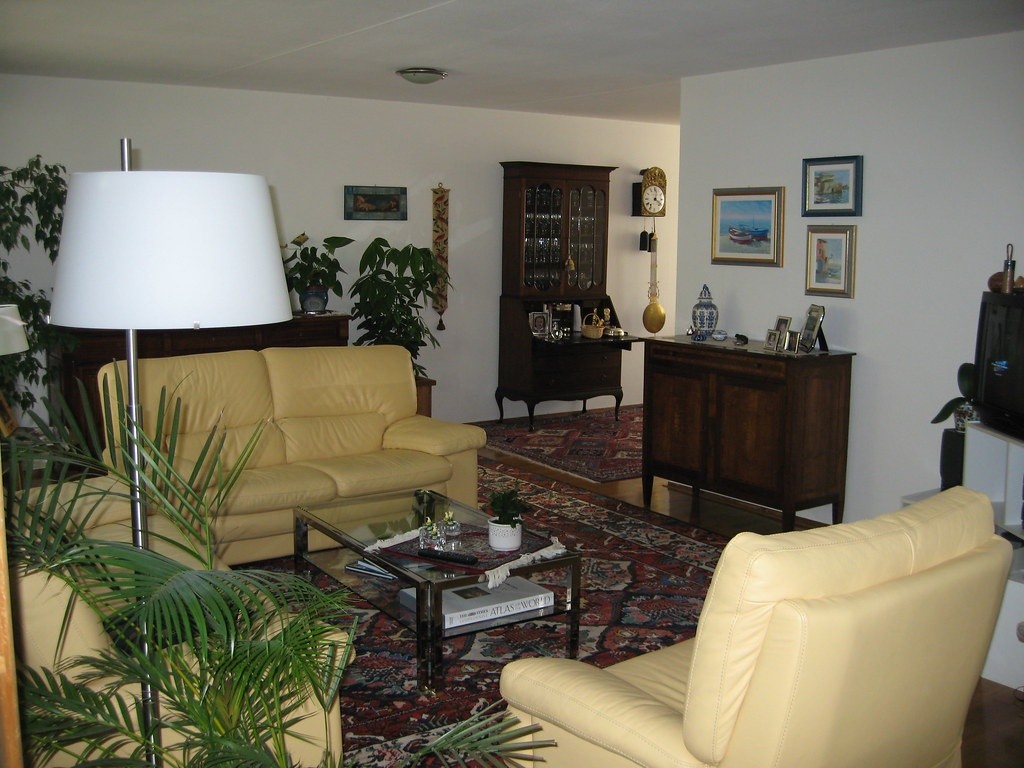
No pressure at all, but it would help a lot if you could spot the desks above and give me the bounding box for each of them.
[1,427,77,473]
[415,375,437,417]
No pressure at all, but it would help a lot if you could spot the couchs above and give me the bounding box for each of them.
[500,485,1013,767]
[3,475,357,768]
[98,345,487,564]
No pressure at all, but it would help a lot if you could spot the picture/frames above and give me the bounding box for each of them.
[775,315,791,351]
[0,392,17,438]
[805,224,857,299]
[788,332,801,354]
[799,304,825,353]
[711,187,785,267]
[533,313,548,334]
[763,329,781,351]
[344,185,407,221]
[801,155,863,217]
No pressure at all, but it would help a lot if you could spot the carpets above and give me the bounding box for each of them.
[364,519,568,590]
[228,455,731,768]
[482,405,643,482]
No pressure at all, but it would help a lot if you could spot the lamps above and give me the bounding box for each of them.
[395,68,448,84]
[50,139,293,768]
[0,304,30,356]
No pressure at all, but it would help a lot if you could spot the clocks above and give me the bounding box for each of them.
[632,166,667,333]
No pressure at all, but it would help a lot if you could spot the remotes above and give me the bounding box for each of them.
[418,548,478,565]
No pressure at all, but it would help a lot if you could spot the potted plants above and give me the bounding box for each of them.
[930,363,976,432]
[480,477,533,552]
[280,232,355,315]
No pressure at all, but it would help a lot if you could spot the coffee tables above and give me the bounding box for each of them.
[292,488,581,691]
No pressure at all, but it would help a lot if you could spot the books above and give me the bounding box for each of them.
[398,575,555,629]
[345,558,397,580]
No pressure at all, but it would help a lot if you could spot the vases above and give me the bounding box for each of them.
[439,520,461,536]
[419,526,446,550]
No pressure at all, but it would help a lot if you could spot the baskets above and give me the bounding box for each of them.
[581,313,604,338]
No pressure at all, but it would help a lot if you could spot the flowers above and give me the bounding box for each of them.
[444,510,454,526]
[424,517,440,540]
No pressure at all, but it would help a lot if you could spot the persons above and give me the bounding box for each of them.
[534,316,545,333]
[768,324,787,346]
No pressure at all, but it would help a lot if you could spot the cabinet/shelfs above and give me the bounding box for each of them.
[901,423,1024,693]
[33,309,353,480]
[639,334,857,531]
[495,161,644,432]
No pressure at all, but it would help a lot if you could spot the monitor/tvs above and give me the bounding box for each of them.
[976,291,1024,441]
